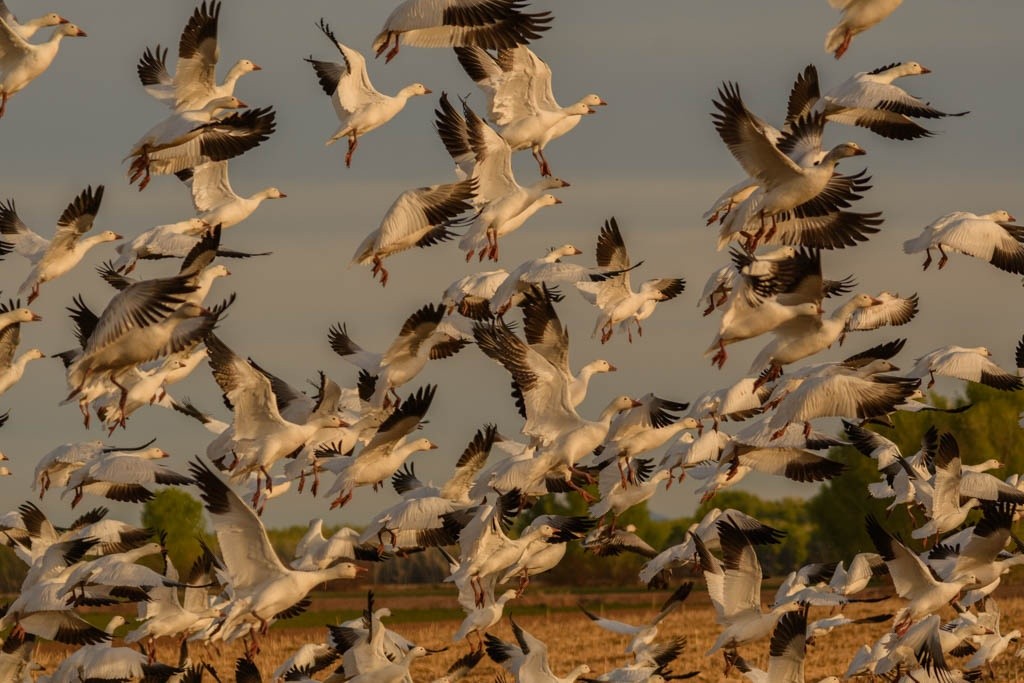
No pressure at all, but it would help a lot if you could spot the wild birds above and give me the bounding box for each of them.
[0,1,1024,683]
